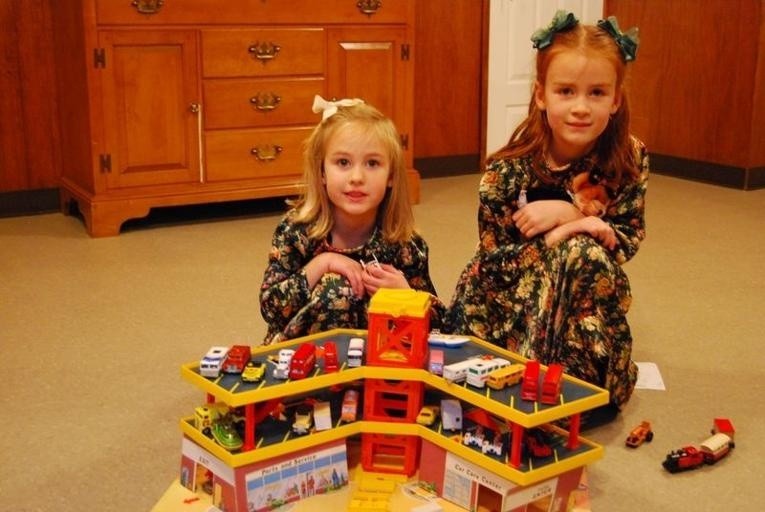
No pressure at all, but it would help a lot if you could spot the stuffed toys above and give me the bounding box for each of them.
[569,165,620,220]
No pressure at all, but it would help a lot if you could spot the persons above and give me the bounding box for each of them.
[256,93,446,339]
[438,8,653,433]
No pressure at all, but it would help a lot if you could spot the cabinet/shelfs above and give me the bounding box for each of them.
[58,1,421,238]
